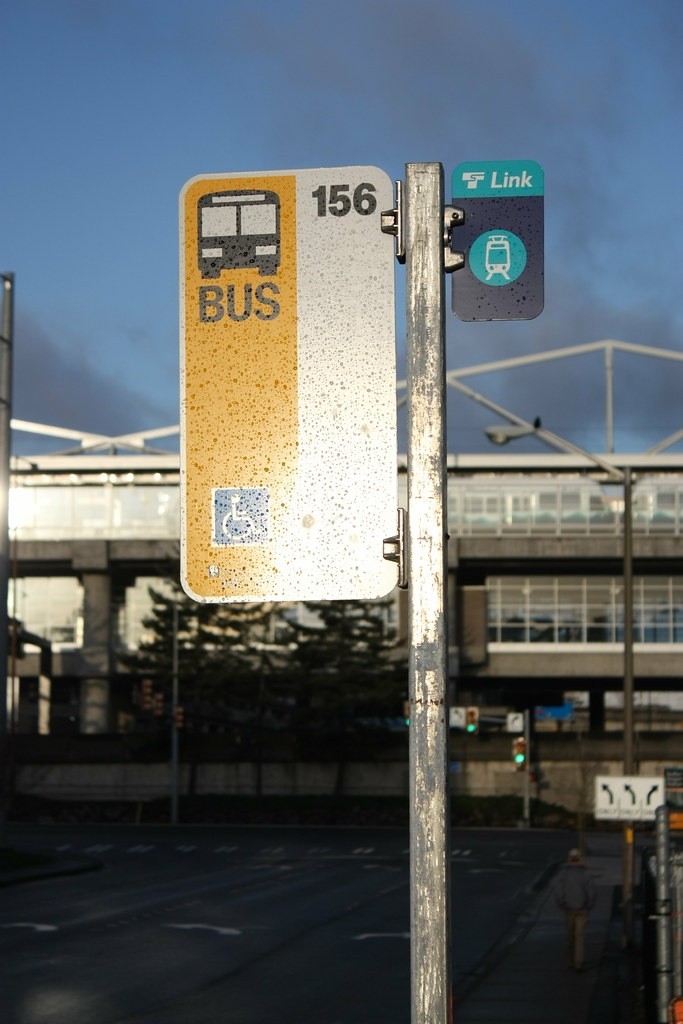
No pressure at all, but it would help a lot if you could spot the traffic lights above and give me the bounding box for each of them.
[404,702,410,726]
[466,706,480,735]
[512,739,527,772]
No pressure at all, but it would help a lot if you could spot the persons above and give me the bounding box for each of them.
[555,848,595,971]
